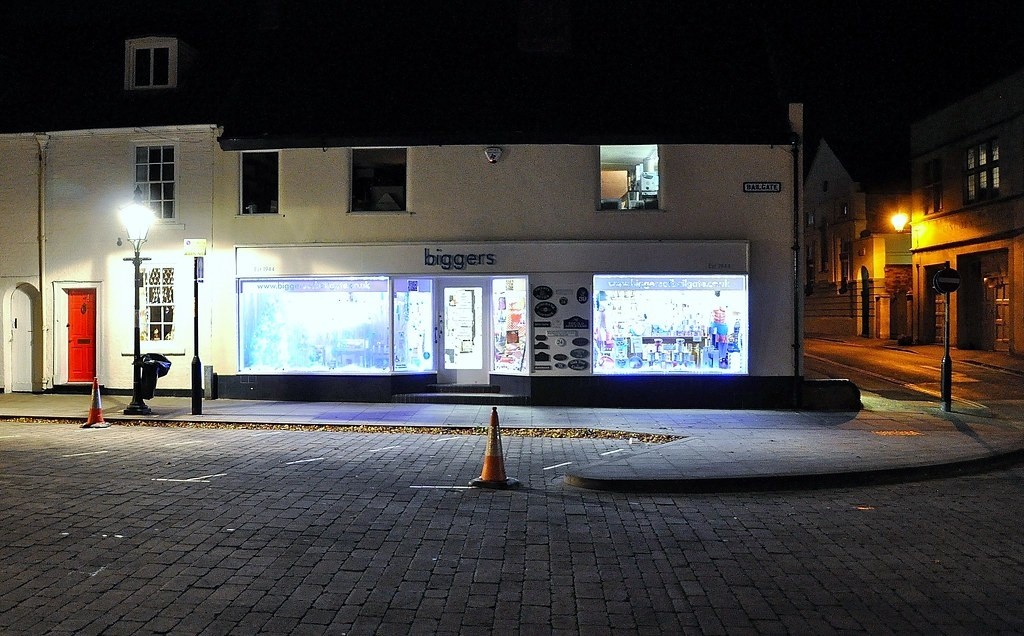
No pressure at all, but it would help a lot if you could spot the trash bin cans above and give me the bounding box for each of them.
[139,353,172,401]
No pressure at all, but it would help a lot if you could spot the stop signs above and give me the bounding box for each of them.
[936,268,962,292]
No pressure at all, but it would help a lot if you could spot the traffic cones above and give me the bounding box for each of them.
[469,407,519,490]
[79,377,111,428]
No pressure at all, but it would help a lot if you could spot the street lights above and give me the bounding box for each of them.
[118,183,156,415]
